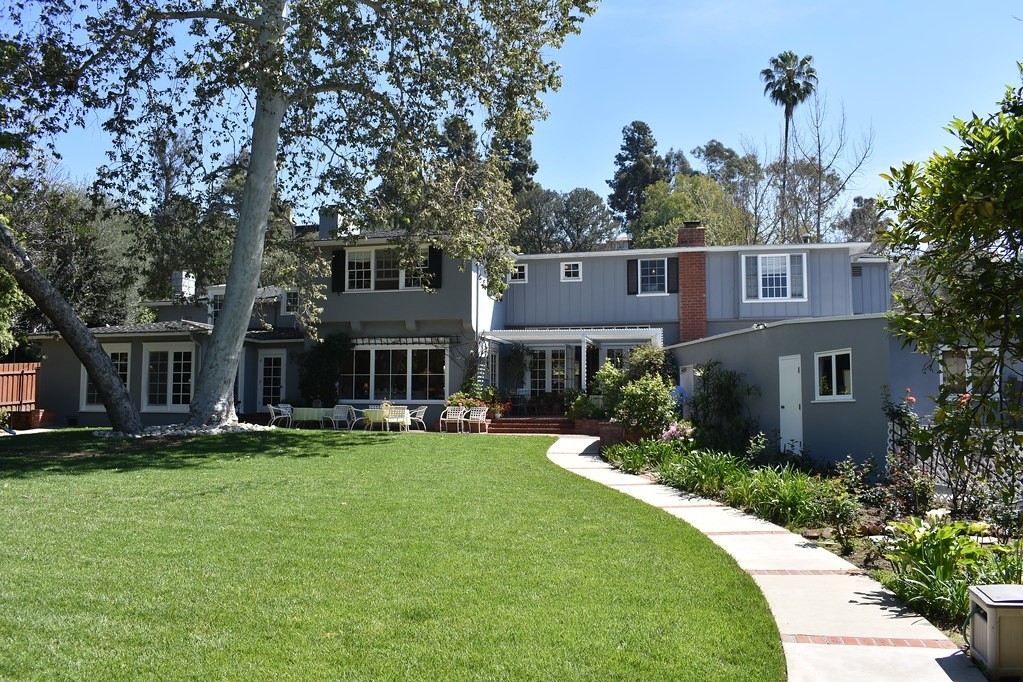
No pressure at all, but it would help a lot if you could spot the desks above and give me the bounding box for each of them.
[363,409,411,431]
[292,408,335,429]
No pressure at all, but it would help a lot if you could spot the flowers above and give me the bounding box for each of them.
[443,388,511,418]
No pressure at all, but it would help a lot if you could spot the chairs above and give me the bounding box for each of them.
[277,403,293,427]
[384,406,407,432]
[347,405,372,431]
[439,406,466,433]
[322,405,349,430]
[267,404,291,428]
[409,406,428,432]
[509,391,554,416]
[461,406,489,433]
[365,405,385,431]
